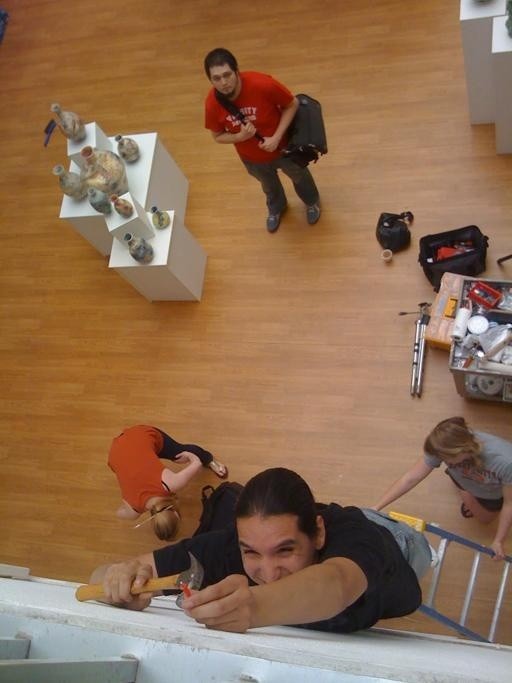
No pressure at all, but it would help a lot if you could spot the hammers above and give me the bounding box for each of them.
[76,550,204,608]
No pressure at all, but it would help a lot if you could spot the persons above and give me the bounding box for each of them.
[106,424,228,541]
[89,467,440,634]
[204,47,321,232]
[369,415,511,561]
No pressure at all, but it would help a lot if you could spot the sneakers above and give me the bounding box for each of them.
[307,202,320,225]
[267,212,280,231]
[428,545,439,569]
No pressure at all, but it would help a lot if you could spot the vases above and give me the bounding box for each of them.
[52,103,171,265]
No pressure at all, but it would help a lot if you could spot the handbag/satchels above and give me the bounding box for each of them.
[376,211,413,250]
[192,480,246,537]
[285,94,327,167]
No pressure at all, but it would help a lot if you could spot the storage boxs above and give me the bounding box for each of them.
[425,272,465,352]
[418,225,489,287]
[448,277,512,403]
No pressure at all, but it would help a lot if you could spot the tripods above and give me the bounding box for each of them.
[411,301,431,395]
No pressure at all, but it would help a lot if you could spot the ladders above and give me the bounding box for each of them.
[385,512,511,644]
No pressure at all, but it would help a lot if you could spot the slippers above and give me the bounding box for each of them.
[461,502,474,517]
[208,461,228,478]
